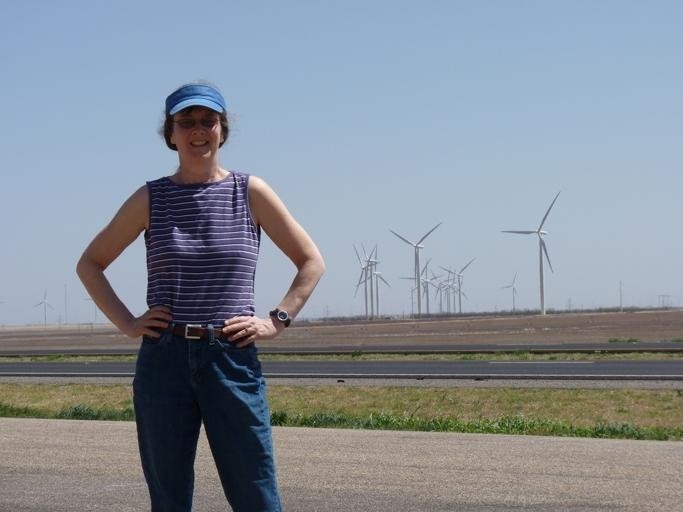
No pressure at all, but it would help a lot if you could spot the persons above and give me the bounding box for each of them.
[74,81,328,512]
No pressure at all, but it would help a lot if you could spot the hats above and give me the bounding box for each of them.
[166,85,225,116]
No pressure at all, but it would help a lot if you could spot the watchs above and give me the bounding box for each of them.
[268,308,291,329]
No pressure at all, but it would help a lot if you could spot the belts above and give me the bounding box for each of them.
[162,323,223,339]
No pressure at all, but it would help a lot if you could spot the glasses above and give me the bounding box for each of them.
[169,118,221,128]
